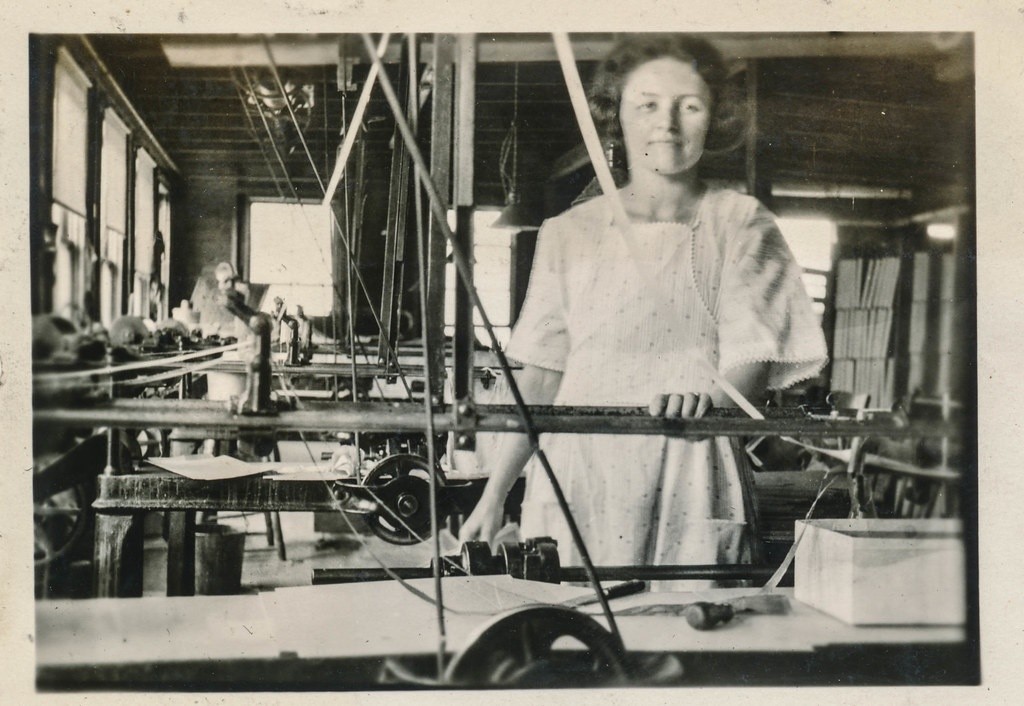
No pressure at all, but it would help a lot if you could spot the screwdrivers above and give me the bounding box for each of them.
[685,602,751,630]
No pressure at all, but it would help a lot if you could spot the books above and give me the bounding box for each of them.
[830,257,905,412]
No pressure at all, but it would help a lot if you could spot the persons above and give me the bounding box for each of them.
[459,32,829,591]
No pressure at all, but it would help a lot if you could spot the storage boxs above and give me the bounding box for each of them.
[795,517,967,629]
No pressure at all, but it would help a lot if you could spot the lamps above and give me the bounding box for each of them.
[573,145,628,206]
[492,62,542,231]
